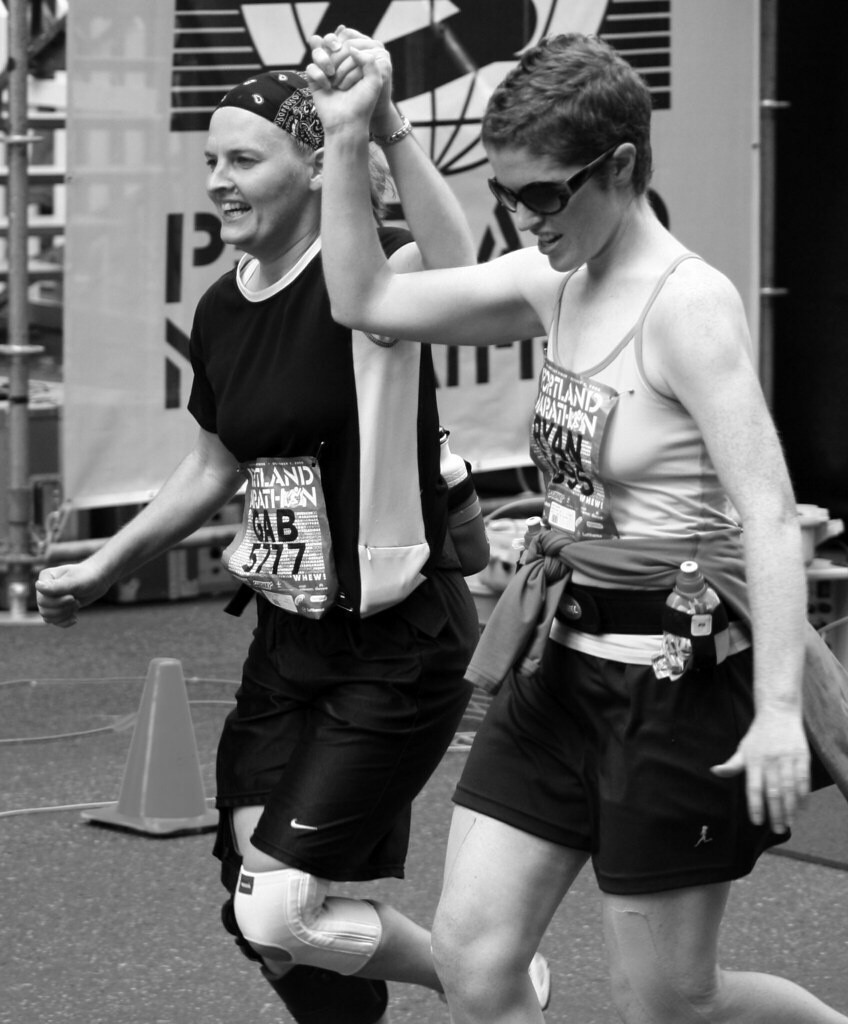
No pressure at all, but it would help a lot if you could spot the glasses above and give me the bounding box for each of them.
[487,144,619,215]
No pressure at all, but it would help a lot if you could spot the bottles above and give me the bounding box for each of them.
[661,560,733,680]
[438,426,467,489]
[523,515,544,549]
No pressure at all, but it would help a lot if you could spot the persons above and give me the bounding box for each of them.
[306,33,848,1024]
[34,25,552,1024]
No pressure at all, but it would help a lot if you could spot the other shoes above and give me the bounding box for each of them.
[527,953,553,1013]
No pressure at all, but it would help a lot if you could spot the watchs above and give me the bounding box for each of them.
[371,115,412,146]
